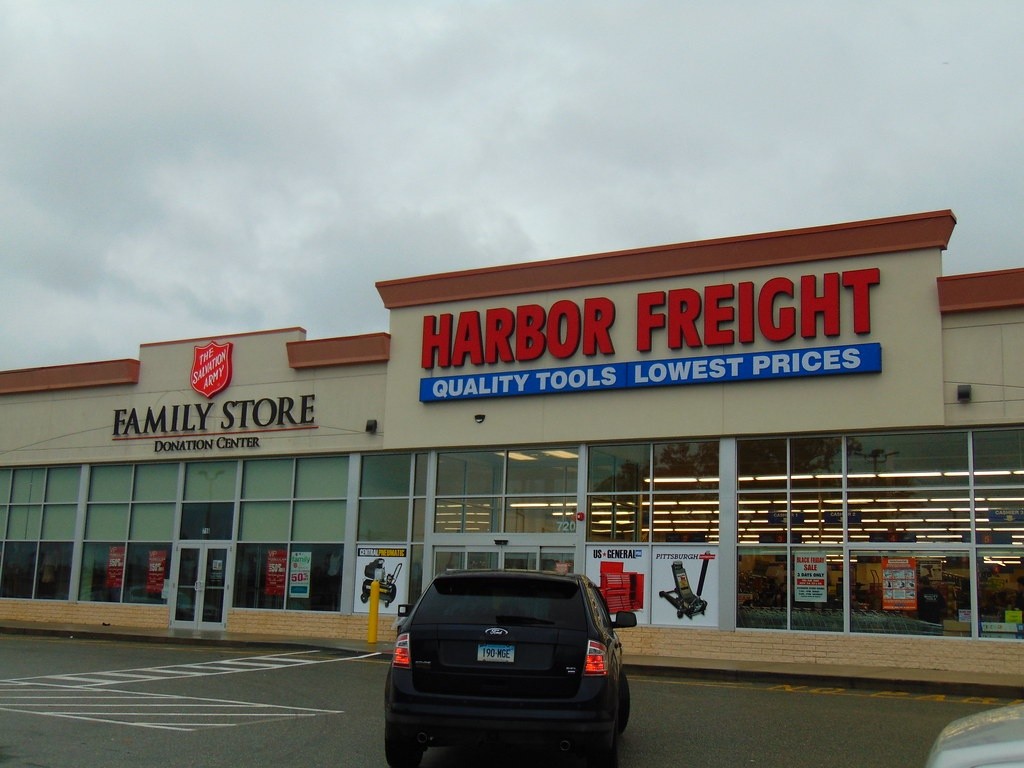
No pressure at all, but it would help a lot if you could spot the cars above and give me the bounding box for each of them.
[23,583,224,622]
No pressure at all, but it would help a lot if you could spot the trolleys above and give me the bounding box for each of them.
[733,606,943,637]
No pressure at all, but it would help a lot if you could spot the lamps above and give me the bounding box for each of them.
[435,449,1024,566]
[957,385,971,401]
[366,419,377,432]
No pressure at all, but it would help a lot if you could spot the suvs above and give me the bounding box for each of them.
[382,570,631,763]
